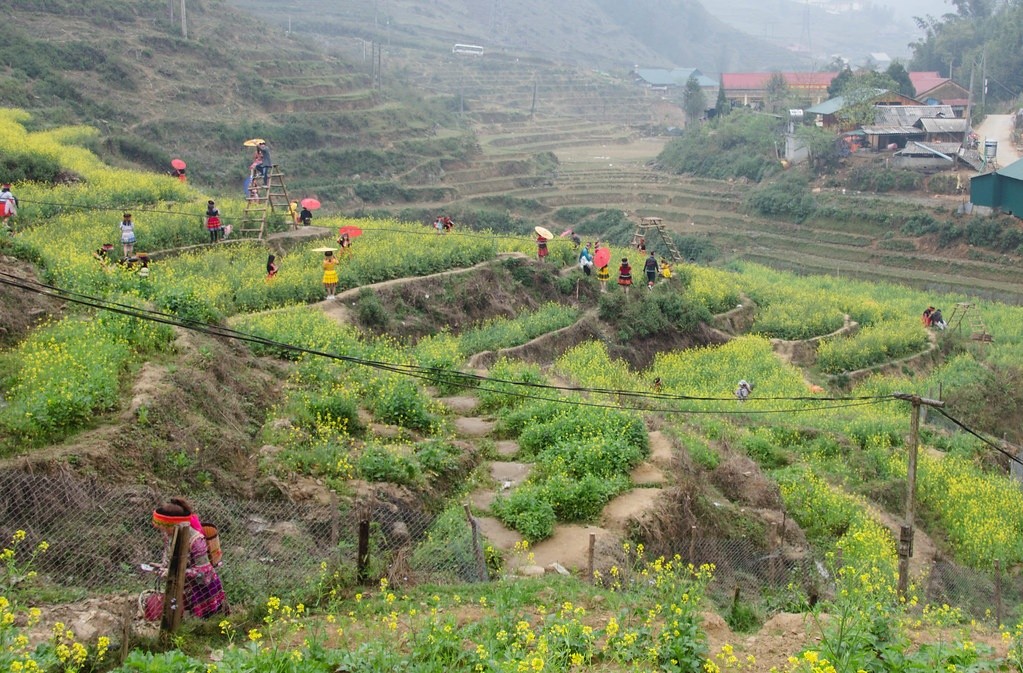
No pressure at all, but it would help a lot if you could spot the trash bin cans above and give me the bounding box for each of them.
[985,140,997,163]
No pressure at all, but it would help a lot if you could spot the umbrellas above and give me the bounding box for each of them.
[311,246,338,254]
[560,228,572,238]
[534,226,553,240]
[300,198,321,211]
[340,225,362,238]
[594,247,610,268]
[171,159,186,170]
[244,139,265,146]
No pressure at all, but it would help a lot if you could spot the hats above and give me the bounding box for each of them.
[930,306,935,308]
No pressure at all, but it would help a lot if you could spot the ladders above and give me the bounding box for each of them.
[241,165,298,238]
[630,217,683,262]
[944,303,993,343]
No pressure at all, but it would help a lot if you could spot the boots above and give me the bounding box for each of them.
[326,285,336,299]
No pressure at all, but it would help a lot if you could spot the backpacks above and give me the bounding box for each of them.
[199,522,222,568]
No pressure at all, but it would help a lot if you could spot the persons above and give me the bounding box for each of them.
[0,183,15,228]
[94,214,150,277]
[206,200,221,245]
[337,232,352,260]
[433,215,454,232]
[536,233,549,263]
[267,255,278,281]
[288,196,312,223]
[746,102,761,112]
[244,143,271,204]
[150,497,231,623]
[736,380,751,402]
[176,169,186,182]
[923,306,947,330]
[321,251,339,300]
[572,232,673,293]
